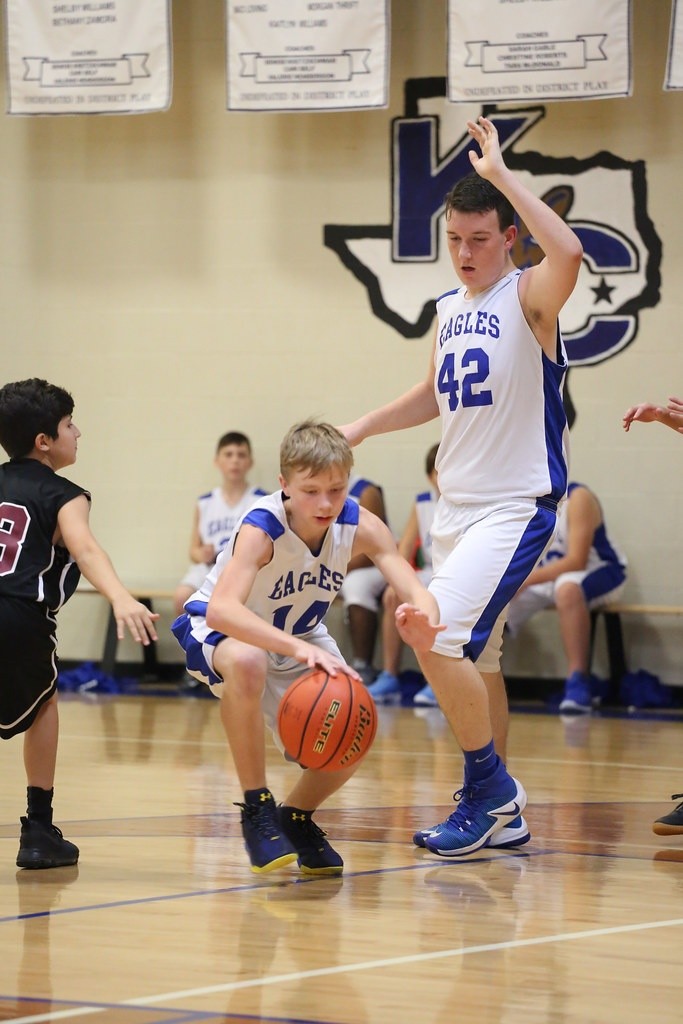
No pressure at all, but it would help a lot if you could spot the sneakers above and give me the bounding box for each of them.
[426,754,528,857]
[15,816,79,868]
[559,670,594,711]
[412,682,439,706]
[16,867,78,910]
[413,813,531,848]
[232,788,299,873]
[276,800,345,874]
[426,862,523,909]
[367,671,403,702]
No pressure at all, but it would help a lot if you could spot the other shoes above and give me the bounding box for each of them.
[652,794,683,836]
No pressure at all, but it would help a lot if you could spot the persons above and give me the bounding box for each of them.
[172,421,448,874]
[622,394,683,836]
[330,116,582,858]
[0,377,160,869]
[508,481,627,712]
[337,470,394,685]
[174,432,270,615]
[364,442,441,705]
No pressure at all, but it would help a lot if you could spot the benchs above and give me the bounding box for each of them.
[70,590,683,706]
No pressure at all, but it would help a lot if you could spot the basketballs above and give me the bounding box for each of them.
[278,668,380,771]
